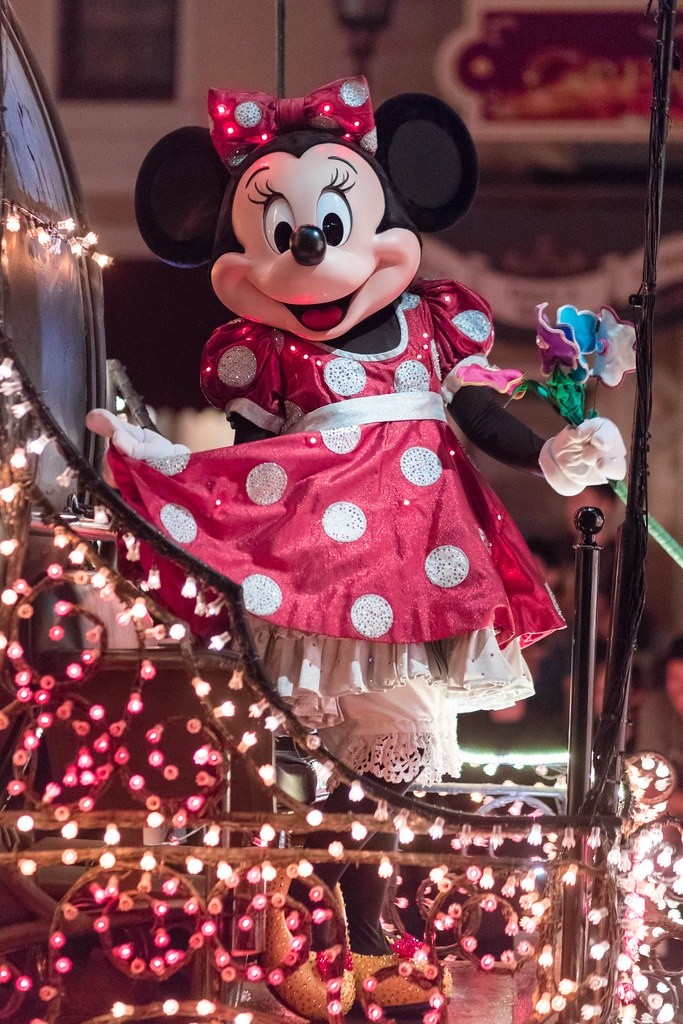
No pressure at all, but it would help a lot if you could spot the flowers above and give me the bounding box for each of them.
[459,299,636,425]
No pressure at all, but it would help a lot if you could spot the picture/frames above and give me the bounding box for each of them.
[55,0,184,108]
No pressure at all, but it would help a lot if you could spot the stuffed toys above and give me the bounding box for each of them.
[86,73,629,1015]
